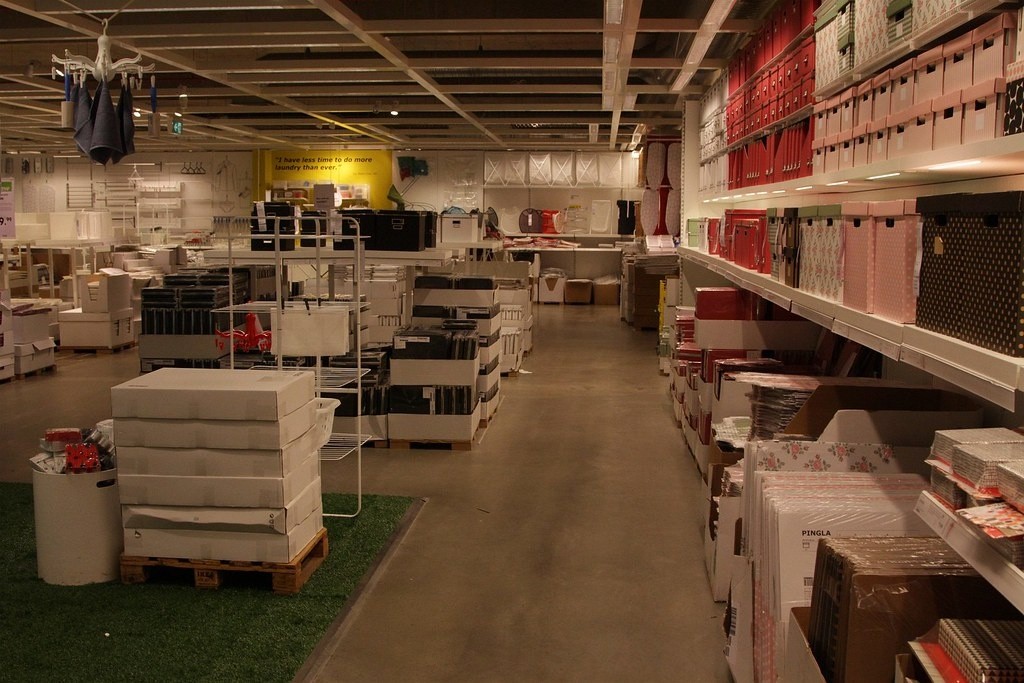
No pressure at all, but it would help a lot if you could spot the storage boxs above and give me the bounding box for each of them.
[594,273,619,306]
[564,277,593,306]
[302,209,439,251]
[537,270,565,305]
[249,200,295,251]
[441,211,480,244]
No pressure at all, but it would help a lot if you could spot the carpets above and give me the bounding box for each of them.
[2,479,429,683]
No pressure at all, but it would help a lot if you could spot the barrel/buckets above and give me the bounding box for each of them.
[31,467,117,585]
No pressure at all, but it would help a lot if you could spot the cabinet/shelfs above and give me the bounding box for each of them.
[682,0,1024,683]
